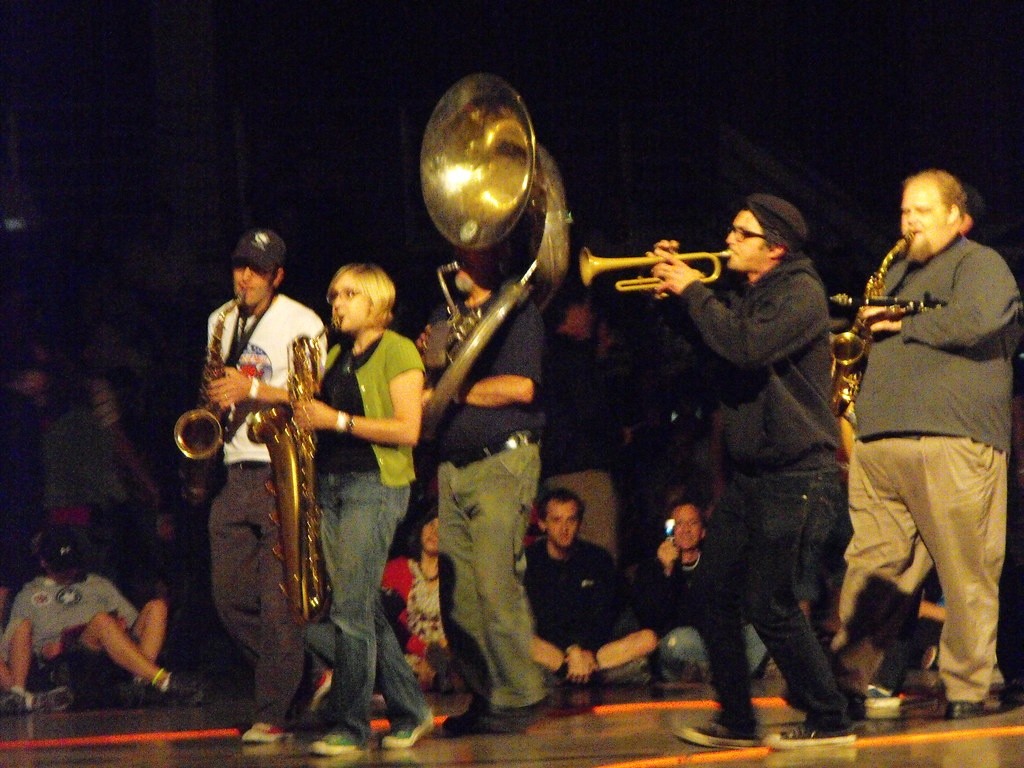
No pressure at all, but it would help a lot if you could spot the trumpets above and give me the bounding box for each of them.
[577,244,734,292]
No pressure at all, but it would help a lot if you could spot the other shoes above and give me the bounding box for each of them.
[442,697,525,737]
[160,671,208,704]
[311,732,358,756]
[0,692,26,715]
[382,707,433,749]
[762,722,857,749]
[672,721,760,748]
[242,722,293,743]
[112,678,147,707]
[945,700,1016,720]
[31,686,72,712]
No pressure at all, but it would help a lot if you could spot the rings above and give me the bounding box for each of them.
[224,393,229,401]
[302,405,307,413]
[306,414,310,422]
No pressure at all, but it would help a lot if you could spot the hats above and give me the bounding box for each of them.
[40,525,91,565]
[745,192,807,253]
[220,229,286,272]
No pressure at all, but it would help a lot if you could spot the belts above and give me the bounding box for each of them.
[449,430,539,469]
[232,460,268,471]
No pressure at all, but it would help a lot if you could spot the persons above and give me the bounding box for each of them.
[199,232,332,742]
[0,280,1024,712]
[416,238,544,736]
[637,193,858,750]
[778,165,1022,720]
[292,262,434,756]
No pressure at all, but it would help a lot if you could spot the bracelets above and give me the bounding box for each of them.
[248,376,259,400]
[336,410,346,433]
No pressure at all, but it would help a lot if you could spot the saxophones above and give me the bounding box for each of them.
[173,287,247,459]
[247,315,350,627]
[828,230,919,417]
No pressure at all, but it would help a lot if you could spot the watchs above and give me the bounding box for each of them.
[346,414,356,433]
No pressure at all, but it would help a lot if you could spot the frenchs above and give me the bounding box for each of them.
[416,73,575,443]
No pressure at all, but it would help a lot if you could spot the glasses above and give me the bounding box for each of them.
[727,222,768,242]
[325,288,361,305]
